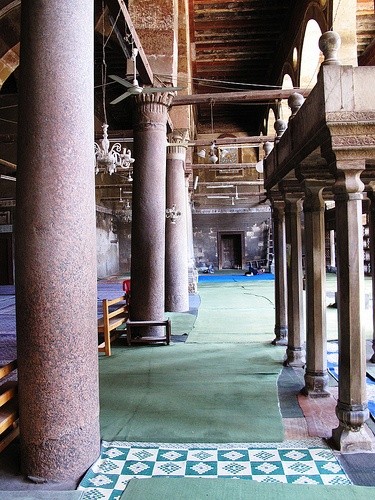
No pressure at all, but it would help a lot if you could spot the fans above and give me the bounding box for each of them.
[109,49,186,105]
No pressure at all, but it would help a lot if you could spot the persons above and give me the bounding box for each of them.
[245,266,265,276]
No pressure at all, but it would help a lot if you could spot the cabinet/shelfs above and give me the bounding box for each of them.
[126,320,172,344]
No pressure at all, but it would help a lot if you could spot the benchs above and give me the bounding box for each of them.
[0,359,20,455]
[98,294,130,356]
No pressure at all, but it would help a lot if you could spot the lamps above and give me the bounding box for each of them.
[197,102,228,163]
[94,62,135,175]
